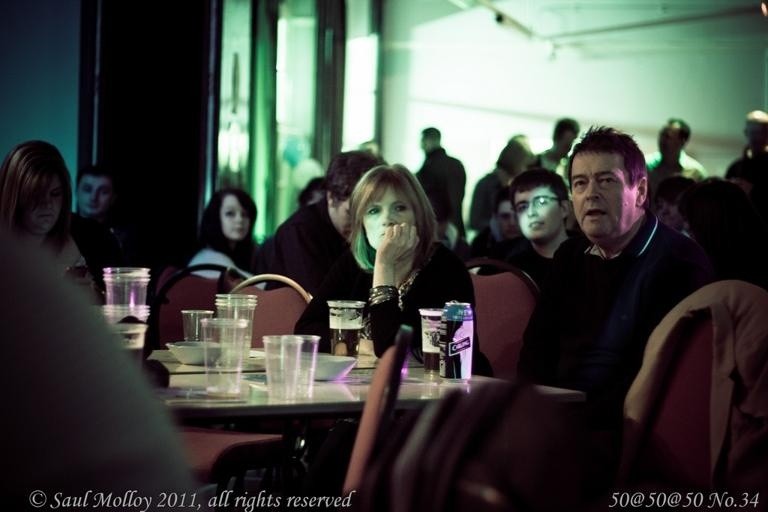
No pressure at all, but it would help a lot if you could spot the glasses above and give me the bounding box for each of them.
[511,195,565,213]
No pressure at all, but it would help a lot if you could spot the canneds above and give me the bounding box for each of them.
[439,300,475,383]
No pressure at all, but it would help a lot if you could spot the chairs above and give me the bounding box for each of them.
[91,254,766,511]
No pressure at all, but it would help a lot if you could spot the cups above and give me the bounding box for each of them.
[418,307,450,371]
[419,373,445,400]
[327,299,367,358]
[101,264,152,372]
[180,292,321,403]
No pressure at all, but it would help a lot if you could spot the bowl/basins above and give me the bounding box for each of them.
[165,342,227,365]
[168,366,219,389]
[310,353,359,383]
[310,381,358,402]
